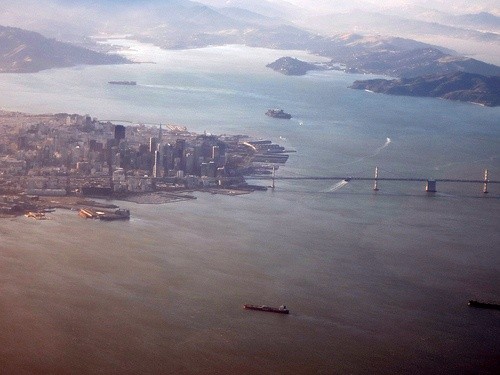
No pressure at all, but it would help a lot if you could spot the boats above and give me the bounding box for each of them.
[79,207,131,220]
[265,108,292,119]
[108,80,136,85]
[344,176,351,182]
[243,302,289,313]
[465,296,500,311]
[27,210,46,221]
